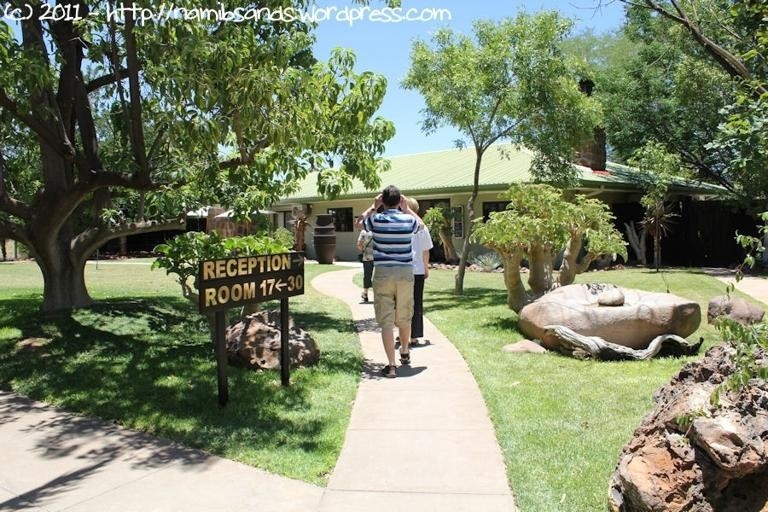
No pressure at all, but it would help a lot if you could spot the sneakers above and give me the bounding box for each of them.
[360,292,369,303]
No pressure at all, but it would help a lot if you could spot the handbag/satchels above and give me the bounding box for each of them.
[357,253,364,263]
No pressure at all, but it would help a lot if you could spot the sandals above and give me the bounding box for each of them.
[382,364,397,378]
[395,336,432,349]
[399,346,412,364]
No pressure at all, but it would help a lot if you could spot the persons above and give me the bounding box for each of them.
[396,194,432,350]
[356,228,374,302]
[353,185,426,378]
